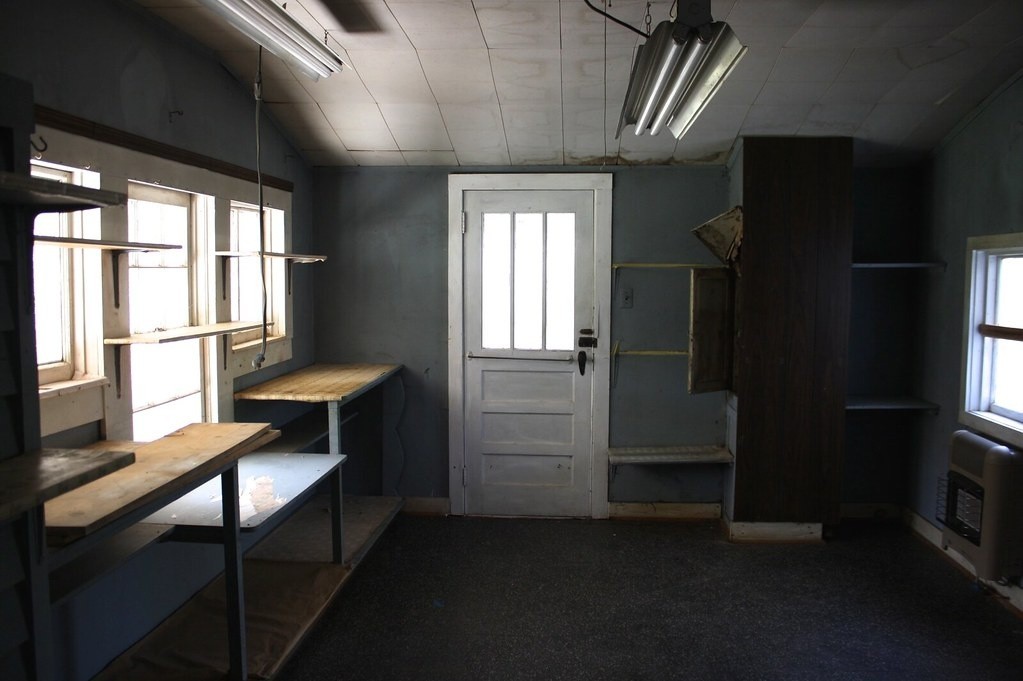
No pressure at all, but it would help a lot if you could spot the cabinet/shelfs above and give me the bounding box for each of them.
[726,136,856,542]
[0,111,50,680]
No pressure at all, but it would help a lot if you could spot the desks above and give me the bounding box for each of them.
[233,363,405,565]
[44,430,280,680]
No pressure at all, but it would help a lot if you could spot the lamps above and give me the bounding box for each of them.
[195,1,343,82]
[614,0,748,142]
